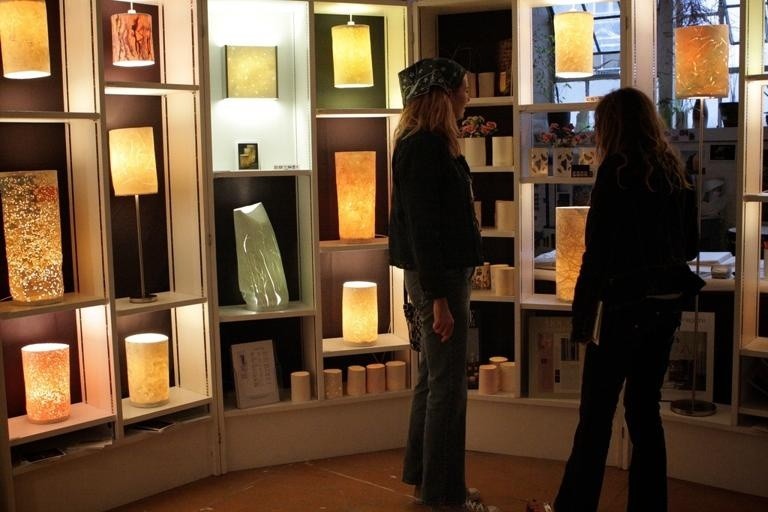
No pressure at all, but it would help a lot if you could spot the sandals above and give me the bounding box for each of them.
[525,497,554,512]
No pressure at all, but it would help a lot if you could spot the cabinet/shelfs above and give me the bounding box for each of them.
[1,0,220,511]
[514,0,632,484]
[202,5,407,487]
[410,2,516,459]
[632,1,768,494]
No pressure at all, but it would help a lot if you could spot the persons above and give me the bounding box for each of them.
[525,86,701,511]
[384,56,503,512]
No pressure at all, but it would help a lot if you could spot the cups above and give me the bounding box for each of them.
[489,263,517,297]
[289,370,312,403]
[497,201,514,231]
[325,361,408,399]
[473,199,484,228]
[477,356,516,396]
[491,135,515,168]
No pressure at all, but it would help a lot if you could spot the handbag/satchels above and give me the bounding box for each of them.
[404,300,474,353]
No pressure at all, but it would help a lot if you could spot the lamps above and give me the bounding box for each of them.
[224,42,281,106]
[670,20,733,419]
[231,201,290,312]
[108,2,158,71]
[547,6,599,82]
[106,122,162,305]
[1,167,68,308]
[329,16,376,92]
[123,331,169,411]
[552,200,588,304]
[340,279,380,345]
[15,341,77,425]
[334,148,379,244]
[3,2,55,82]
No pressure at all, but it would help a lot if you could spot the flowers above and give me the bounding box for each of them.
[539,117,592,147]
[459,112,497,138]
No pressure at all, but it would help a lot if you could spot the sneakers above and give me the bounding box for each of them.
[424,497,502,512]
[412,482,481,504]
